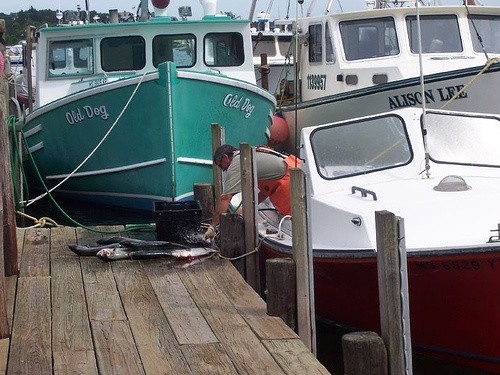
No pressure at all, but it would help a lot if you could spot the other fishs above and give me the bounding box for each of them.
[67,235,219,260]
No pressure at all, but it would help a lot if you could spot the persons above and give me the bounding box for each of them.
[201,144,301,243]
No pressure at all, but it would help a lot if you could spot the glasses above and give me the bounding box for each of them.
[218,152,227,169]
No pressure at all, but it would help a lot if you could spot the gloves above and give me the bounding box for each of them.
[202,224,215,243]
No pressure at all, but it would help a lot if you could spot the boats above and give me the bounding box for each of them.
[7,0,500,375]
[7,0,278,215]
